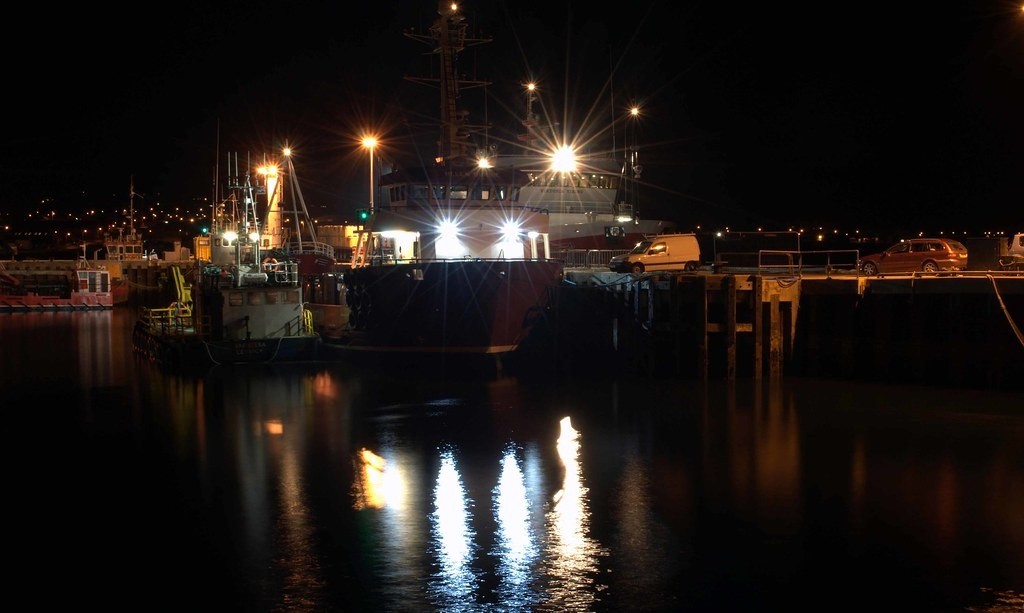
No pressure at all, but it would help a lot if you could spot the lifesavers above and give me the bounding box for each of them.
[132,327,186,372]
[346,289,374,311]
[262,257,278,272]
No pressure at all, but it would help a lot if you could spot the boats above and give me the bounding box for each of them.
[100,174,146,262]
[301,0,567,374]
[132,117,352,372]
[71,241,130,306]
[376,49,679,266]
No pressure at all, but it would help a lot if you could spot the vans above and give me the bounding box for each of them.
[607,232,702,274]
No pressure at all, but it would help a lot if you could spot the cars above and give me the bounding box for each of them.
[1000,232,1024,269]
[854,238,968,276]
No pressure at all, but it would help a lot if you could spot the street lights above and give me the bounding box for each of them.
[623,105,640,204]
[713,231,723,264]
[359,136,379,265]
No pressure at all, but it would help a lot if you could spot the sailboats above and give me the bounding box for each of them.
[258,138,338,274]
